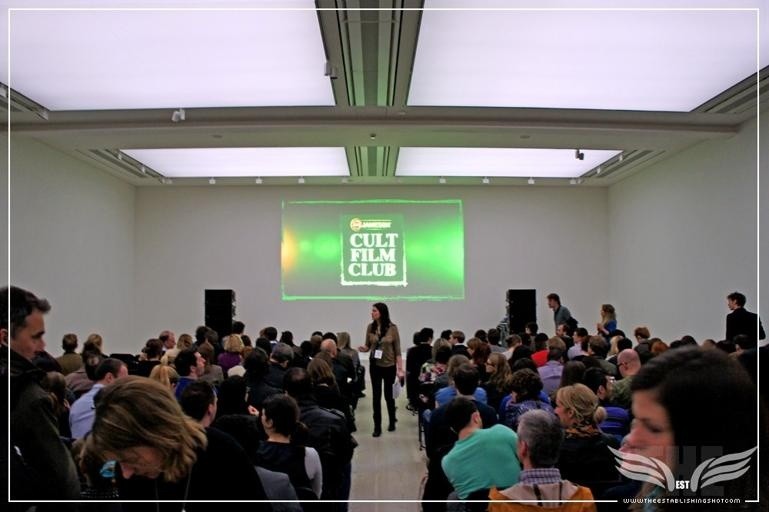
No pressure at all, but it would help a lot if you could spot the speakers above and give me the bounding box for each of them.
[204,288,236,344]
[505,288,537,333]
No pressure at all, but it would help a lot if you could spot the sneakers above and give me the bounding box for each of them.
[388,425,395,431]
[373,430,381,436]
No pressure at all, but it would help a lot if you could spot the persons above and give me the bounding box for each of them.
[358,303,405,438]
[406,327,434,410]
[0,286,79,511]
[220,321,358,363]
[441,398,519,499]
[670,335,756,347]
[426,364,497,430]
[177,380,245,452]
[81,333,105,354]
[92,376,275,512]
[139,327,220,376]
[67,357,130,440]
[180,381,249,458]
[412,333,421,345]
[588,336,617,374]
[536,348,565,397]
[432,328,524,360]
[583,367,625,408]
[521,293,587,366]
[434,354,488,409]
[596,304,616,336]
[488,409,597,512]
[503,368,556,427]
[245,393,323,505]
[726,291,766,346]
[610,327,666,366]
[554,383,624,481]
[56,332,80,374]
[479,353,513,387]
[627,346,756,512]
[512,358,539,374]
[306,357,357,433]
[281,367,350,450]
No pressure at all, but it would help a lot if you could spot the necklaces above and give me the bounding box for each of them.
[155,469,192,512]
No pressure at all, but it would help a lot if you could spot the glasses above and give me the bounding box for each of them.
[617,362,628,368]
